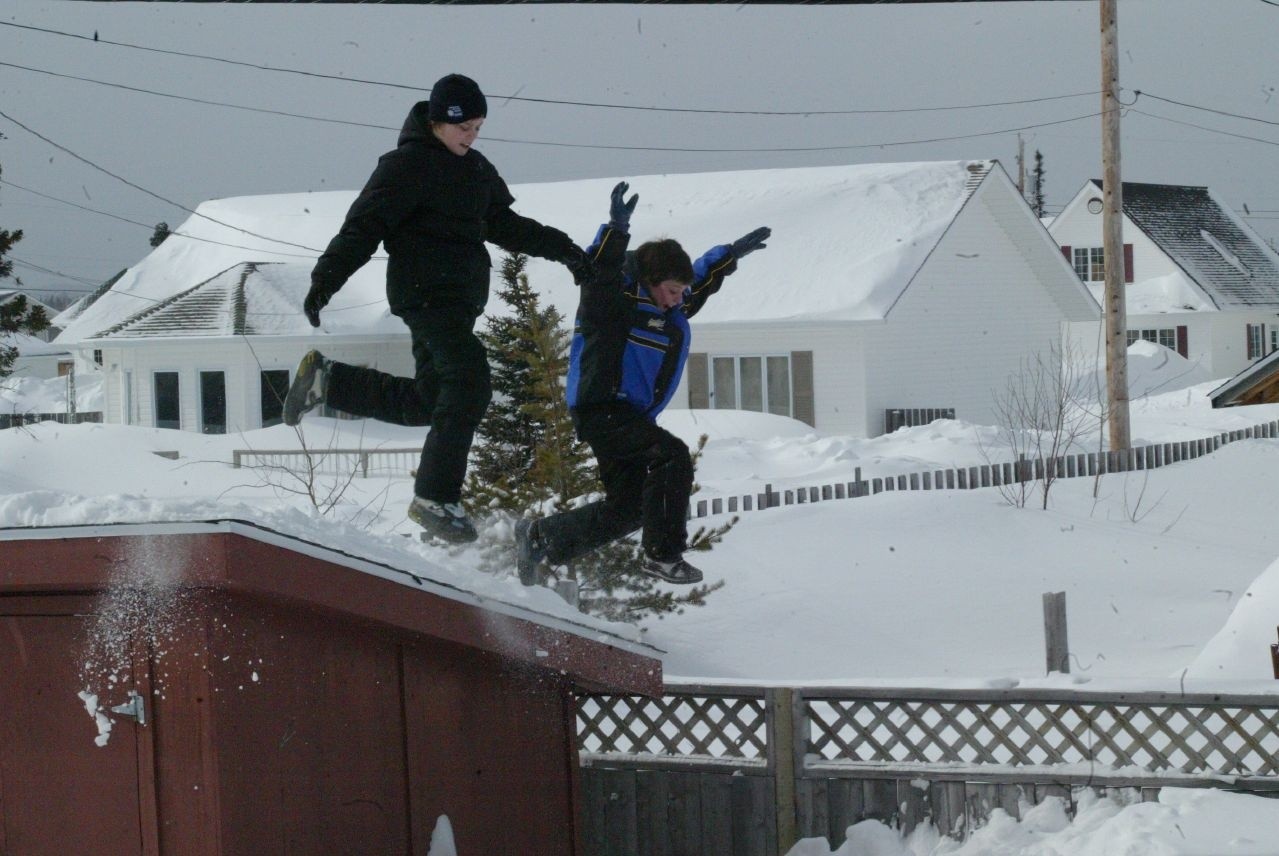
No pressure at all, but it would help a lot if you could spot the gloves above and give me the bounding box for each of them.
[562,245,601,289]
[304,279,334,329]
[608,180,639,234]
[734,226,772,257]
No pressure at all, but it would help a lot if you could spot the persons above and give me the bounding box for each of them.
[307,74,596,545]
[513,182,771,587]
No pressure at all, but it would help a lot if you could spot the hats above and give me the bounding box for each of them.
[428,76,487,123]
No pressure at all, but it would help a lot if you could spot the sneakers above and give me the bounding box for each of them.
[284,348,333,426]
[408,495,480,544]
[641,553,703,585]
[515,514,547,586]
